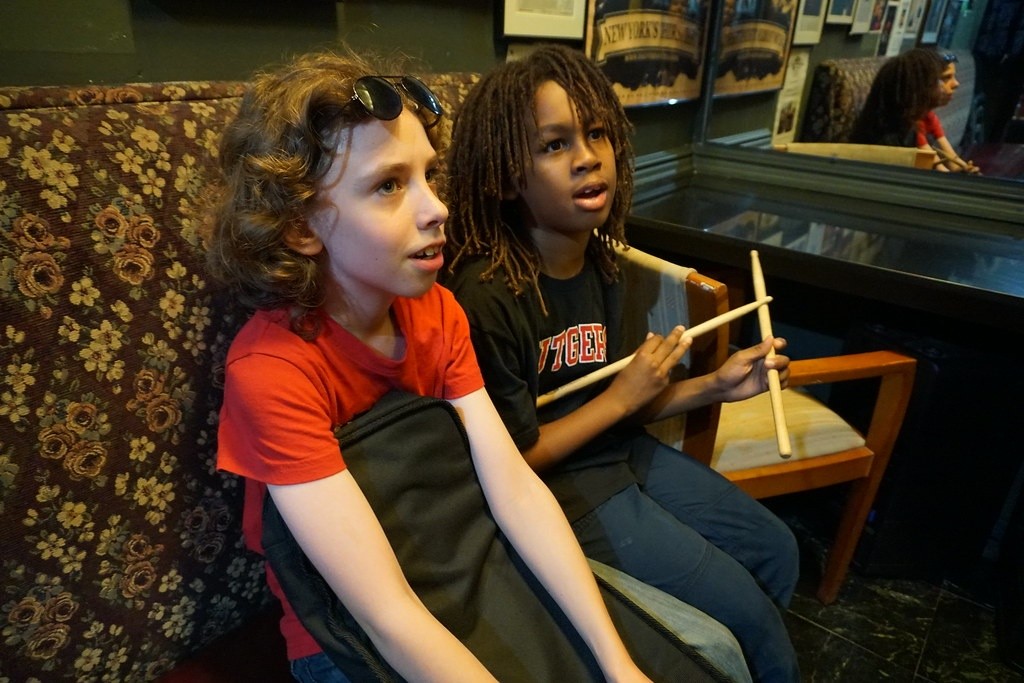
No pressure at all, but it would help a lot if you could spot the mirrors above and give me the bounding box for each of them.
[695,0,1024,226]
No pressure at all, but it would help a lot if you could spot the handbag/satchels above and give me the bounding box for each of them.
[260,388,609,683]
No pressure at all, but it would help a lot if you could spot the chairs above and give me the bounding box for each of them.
[590,226,925,606]
[801,48,1024,181]
[0,70,482,683]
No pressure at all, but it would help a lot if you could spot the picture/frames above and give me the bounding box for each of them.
[490,0,712,124]
[714,0,951,104]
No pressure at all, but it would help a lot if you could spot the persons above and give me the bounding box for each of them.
[211,40,754,683]
[846,45,983,177]
[447,37,808,683]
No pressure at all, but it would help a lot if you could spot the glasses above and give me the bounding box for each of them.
[941,55,959,66]
[317,74,444,135]
